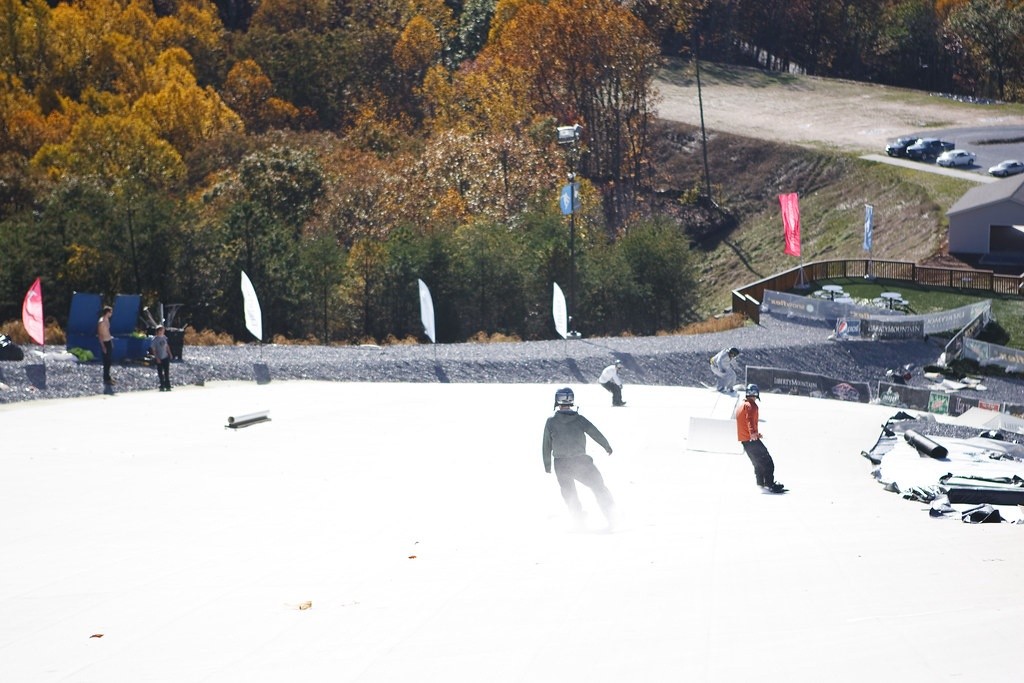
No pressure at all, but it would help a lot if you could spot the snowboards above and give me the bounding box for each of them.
[761,484,787,494]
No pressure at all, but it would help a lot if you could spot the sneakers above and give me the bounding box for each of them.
[613,402,627,407]
[719,389,737,395]
[758,483,787,495]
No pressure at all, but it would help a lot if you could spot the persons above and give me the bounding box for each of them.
[542,388,612,502]
[98,305,117,386]
[599,360,627,407]
[709,347,741,393]
[150,325,173,393]
[735,384,787,493]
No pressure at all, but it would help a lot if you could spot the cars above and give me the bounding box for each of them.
[989,159,1024,177]
[936,150,977,168]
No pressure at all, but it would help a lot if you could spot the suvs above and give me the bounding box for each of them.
[886,137,920,157]
[907,139,955,160]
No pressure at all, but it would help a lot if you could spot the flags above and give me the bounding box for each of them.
[778,192,802,258]
[417,278,436,344]
[863,205,872,252]
[240,270,263,339]
[552,282,568,339]
[22,277,45,345]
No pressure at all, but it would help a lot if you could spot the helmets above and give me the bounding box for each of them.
[730,348,739,356]
[615,360,622,364]
[554,387,575,407]
[746,384,759,397]
